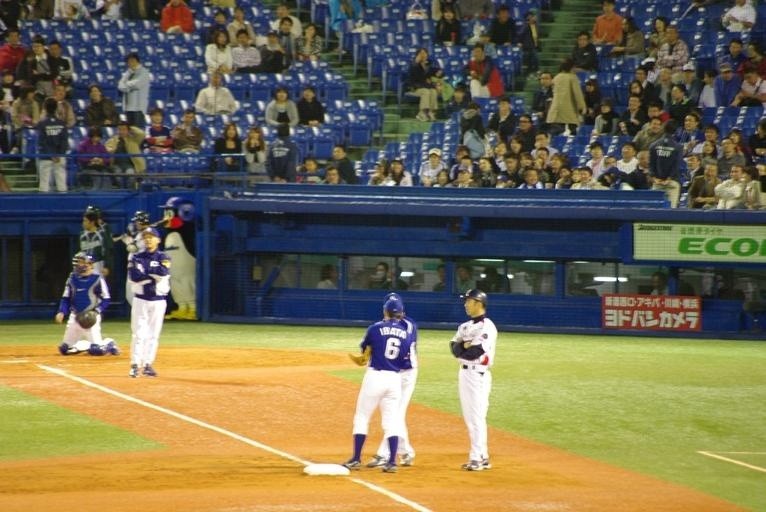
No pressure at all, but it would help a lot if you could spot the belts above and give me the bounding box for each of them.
[462,364,475,369]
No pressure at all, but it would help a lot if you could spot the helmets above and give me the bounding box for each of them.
[131,210,151,223]
[460,287,489,307]
[86,205,101,218]
[72,248,97,278]
[384,293,402,305]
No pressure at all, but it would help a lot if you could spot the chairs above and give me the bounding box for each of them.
[1,1,766,208]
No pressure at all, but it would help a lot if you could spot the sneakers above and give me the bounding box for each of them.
[382,462,398,473]
[462,458,491,471]
[142,364,157,377]
[368,453,387,468]
[342,460,361,469]
[107,337,121,355]
[468,460,484,471]
[128,363,139,377]
[427,112,436,121]
[415,112,428,122]
[401,453,413,466]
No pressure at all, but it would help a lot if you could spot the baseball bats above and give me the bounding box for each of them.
[111,219,168,242]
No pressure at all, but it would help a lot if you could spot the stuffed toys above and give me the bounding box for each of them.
[153,196,200,320]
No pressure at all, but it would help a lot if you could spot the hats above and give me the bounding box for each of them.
[683,64,695,72]
[142,226,159,238]
[373,148,473,176]
[382,300,404,314]
[721,62,734,73]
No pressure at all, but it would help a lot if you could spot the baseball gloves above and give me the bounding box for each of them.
[348,346,372,367]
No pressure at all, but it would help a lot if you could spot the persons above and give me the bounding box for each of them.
[450,286,498,473]
[125,226,175,379]
[54,251,122,357]
[343,299,411,474]
[80,205,154,278]
[266,255,766,322]
[366,292,415,469]
[0,0,766,210]
